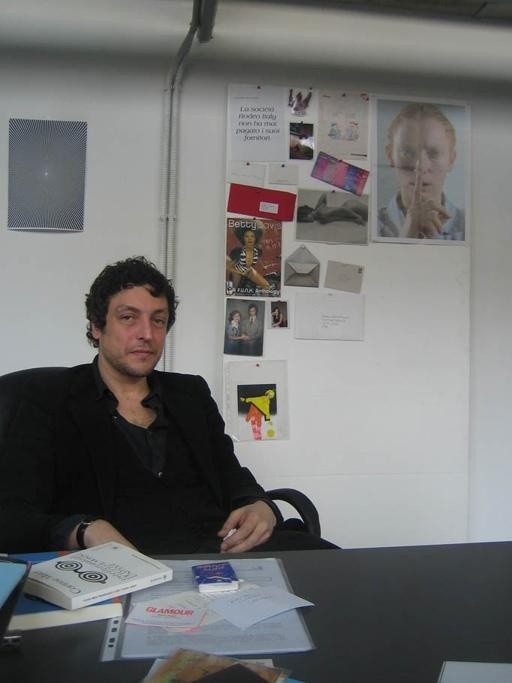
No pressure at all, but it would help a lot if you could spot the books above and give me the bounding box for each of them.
[23,541,173,611]
[138,647,302,683]
[7,549,124,630]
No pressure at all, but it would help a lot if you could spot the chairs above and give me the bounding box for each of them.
[0,367,321,556]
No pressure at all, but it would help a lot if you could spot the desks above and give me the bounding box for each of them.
[1,541,512,679]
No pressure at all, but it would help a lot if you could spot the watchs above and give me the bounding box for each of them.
[75,512,100,551]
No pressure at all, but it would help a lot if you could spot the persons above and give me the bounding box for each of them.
[270,302,286,327]
[224,220,273,296]
[224,301,262,356]
[239,390,275,440]
[290,123,314,159]
[377,102,467,241]
[288,88,314,115]
[297,192,369,225]
[0,255,281,554]
